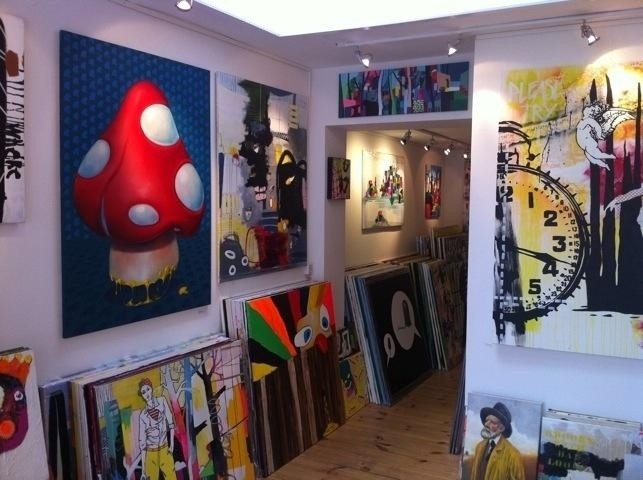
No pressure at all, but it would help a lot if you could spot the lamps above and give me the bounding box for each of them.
[174,0,194,12]
[399,129,470,161]
[576,18,602,47]
[353,46,375,69]
[446,37,462,57]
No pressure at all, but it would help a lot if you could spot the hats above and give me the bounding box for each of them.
[480,402,512,438]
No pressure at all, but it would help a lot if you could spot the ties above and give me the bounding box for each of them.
[480,441,497,479]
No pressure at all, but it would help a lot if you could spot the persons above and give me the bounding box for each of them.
[605,184,643,212]
[576,100,616,171]
[136,377,177,480]
[470,402,526,480]
